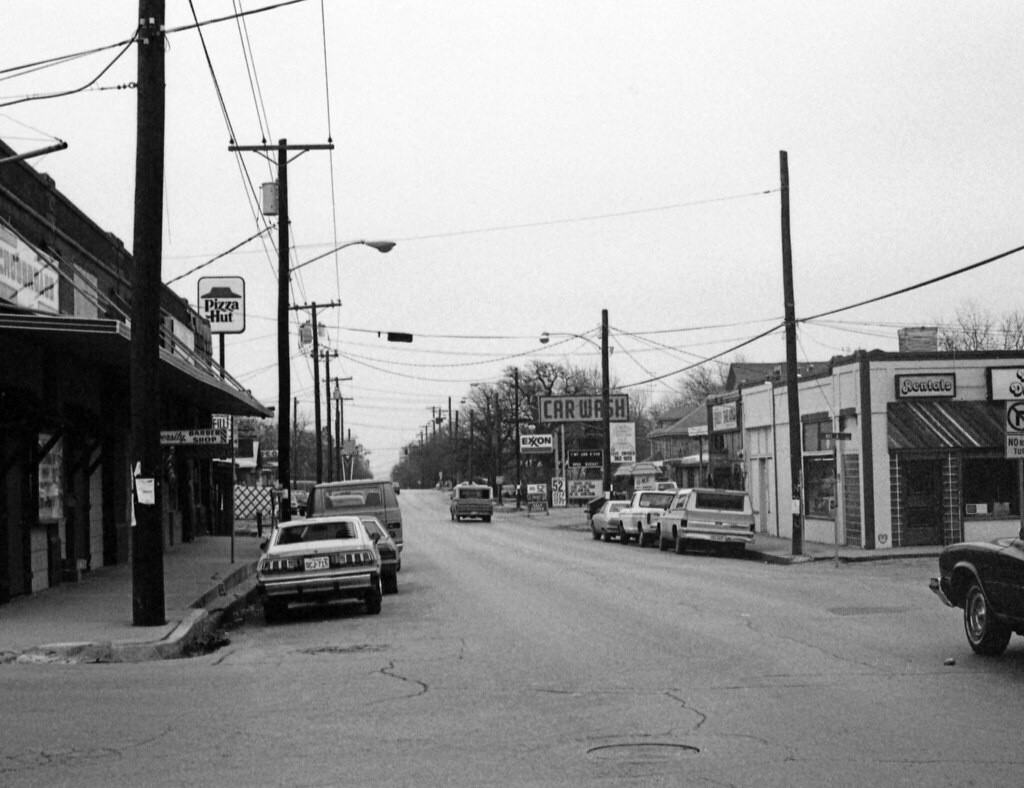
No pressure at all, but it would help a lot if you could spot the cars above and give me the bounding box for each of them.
[300,515,403,594]
[618,489,675,546]
[591,499,631,542]
[255,514,384,616]
[927,538,1024,654]
[655,487,756,554]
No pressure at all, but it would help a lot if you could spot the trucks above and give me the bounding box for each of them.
[450,483,495,522]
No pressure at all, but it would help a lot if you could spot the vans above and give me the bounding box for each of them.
[305,476,404,553]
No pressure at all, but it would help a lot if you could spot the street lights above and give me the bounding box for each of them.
[278,240,399,520]
[539,309,612,492]
